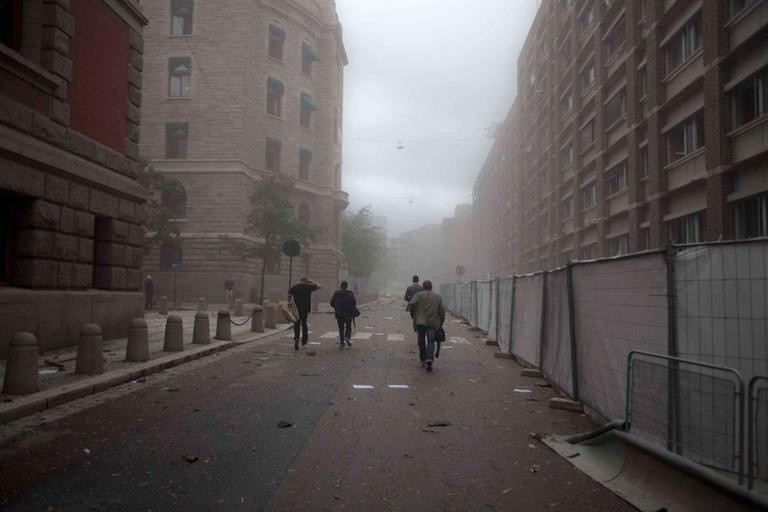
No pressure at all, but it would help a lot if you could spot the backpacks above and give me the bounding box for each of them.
[278,300,300,322]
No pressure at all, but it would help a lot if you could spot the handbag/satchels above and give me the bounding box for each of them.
[435,329,445,342]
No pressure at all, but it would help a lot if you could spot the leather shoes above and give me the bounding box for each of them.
[421,359,432,372]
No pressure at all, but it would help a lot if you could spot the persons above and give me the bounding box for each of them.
[144,275,154,310]
[288,276,323,351]
[405,274,445,372]
[330,281,361,349]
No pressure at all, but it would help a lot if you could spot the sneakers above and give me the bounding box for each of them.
[345,337,352,347]
[295,337,298,350]
